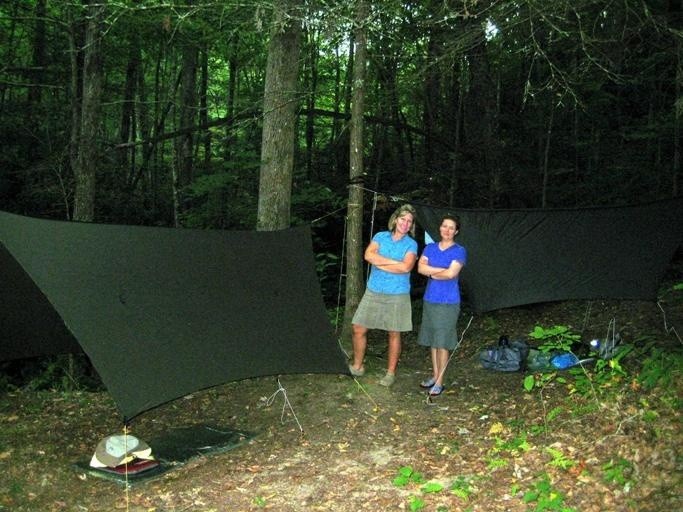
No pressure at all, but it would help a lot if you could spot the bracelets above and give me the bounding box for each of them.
[429,275,432,279]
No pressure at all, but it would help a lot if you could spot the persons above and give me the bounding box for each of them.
[416,215,467,396]
[348,204,418,386]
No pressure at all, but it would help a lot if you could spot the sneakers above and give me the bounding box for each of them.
[378,372,394,387]
[348,365,364,376]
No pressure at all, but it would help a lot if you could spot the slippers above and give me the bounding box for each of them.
[419,377,434,389]
[426,384,444,396]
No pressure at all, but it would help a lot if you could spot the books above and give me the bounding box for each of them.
[97,459,160,476]
